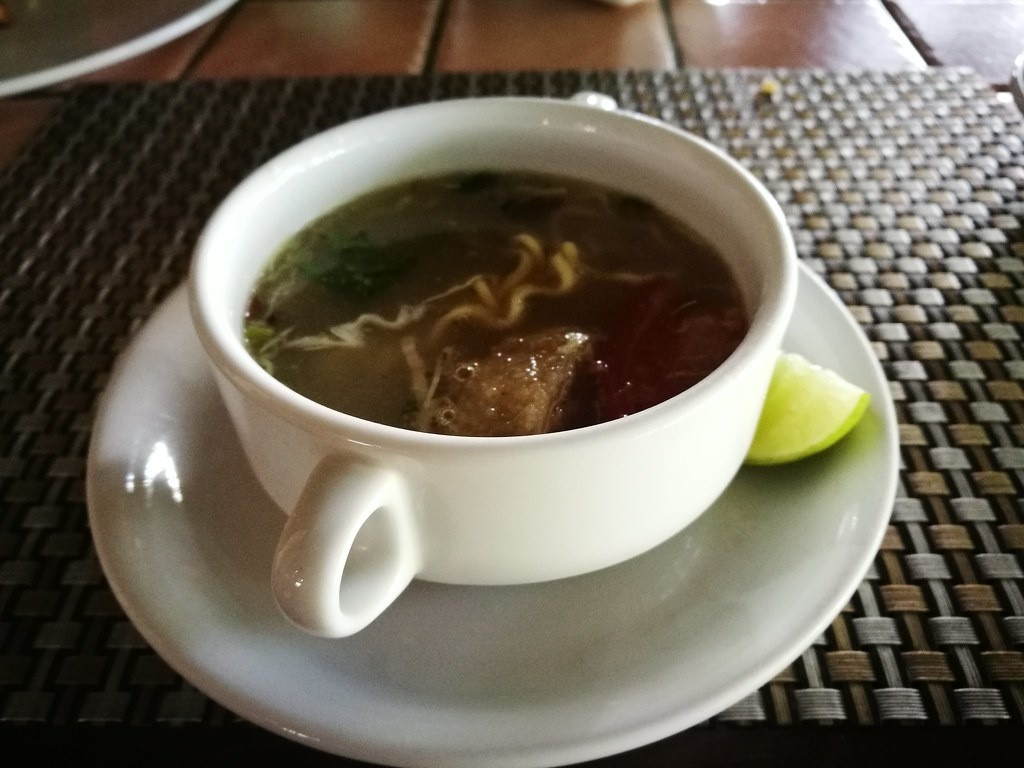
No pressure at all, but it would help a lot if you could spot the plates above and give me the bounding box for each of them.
[86,254,897,768]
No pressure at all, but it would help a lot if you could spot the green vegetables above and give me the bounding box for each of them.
[292,229,387,300]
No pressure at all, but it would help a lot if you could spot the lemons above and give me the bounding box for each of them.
[742,351,870,462]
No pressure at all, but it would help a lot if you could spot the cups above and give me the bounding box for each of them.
[187,100,796,639]
